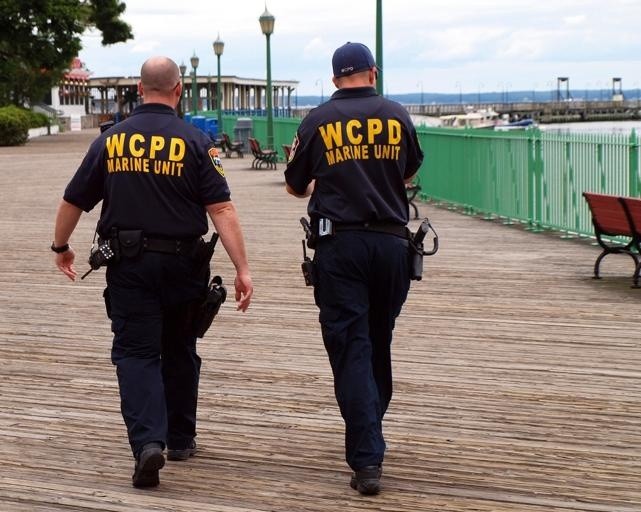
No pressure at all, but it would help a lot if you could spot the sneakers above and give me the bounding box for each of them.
[349,464,384,498]
[131,445,168,489]
[164,437,200,462]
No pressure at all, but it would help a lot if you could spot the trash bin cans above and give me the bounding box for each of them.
[183,112,219,140]
[100,121,114,134]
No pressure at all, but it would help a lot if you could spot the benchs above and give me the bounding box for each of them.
[247,137,276,170]
[223,133,244,158]
[406,174,421,219]
[582,191,641,289]
[281,144,291,160]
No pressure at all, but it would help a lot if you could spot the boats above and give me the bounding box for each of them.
[439,107,533,129]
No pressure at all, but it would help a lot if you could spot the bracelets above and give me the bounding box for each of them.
[51,241,72,257]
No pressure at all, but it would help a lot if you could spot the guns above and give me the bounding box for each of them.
[413,222,429,246]
[209,287,224,307]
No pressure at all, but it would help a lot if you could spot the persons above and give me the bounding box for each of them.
[281,38,425,498]
[50,55,255,490]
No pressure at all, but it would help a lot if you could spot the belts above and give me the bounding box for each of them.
[327,222,412,241]
[142,239,184,254]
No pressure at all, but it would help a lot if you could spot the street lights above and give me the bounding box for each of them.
[179,61,187,113]
[213,35,225,132]
[191,51,199,116]
[258,0,275,151]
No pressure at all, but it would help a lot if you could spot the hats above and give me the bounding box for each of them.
[331,41,383,78]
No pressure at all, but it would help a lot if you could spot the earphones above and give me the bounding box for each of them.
[375,71,379,79]
